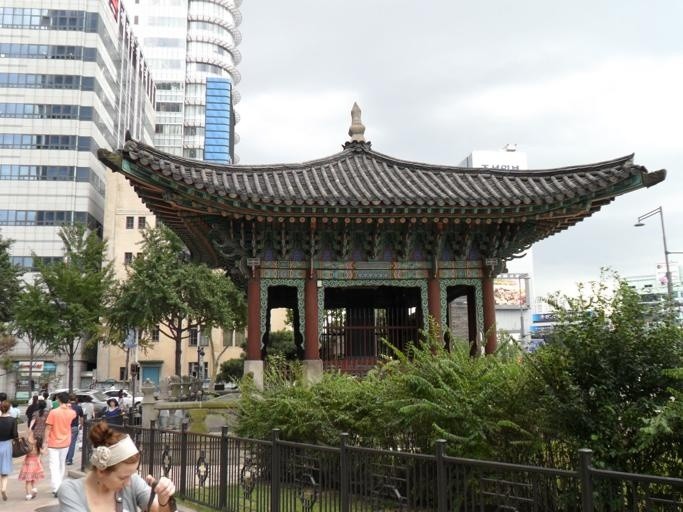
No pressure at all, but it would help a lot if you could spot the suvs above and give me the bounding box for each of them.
[44,387,143,418]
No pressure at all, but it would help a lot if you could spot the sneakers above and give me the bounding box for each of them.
[26,488,37,500]
[2,489,7,500]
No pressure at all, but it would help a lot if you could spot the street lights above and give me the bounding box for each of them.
[517,273,529,347]
[195,345,205,379]
[130,360,139,409]
[634,205,677,328]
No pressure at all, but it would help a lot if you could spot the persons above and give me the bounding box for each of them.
[0,384,124,501]
[56,420,176,512]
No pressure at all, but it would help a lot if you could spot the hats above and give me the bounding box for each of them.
[107,398,118,406]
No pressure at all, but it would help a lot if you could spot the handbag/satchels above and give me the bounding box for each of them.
[13,437,32,457]
